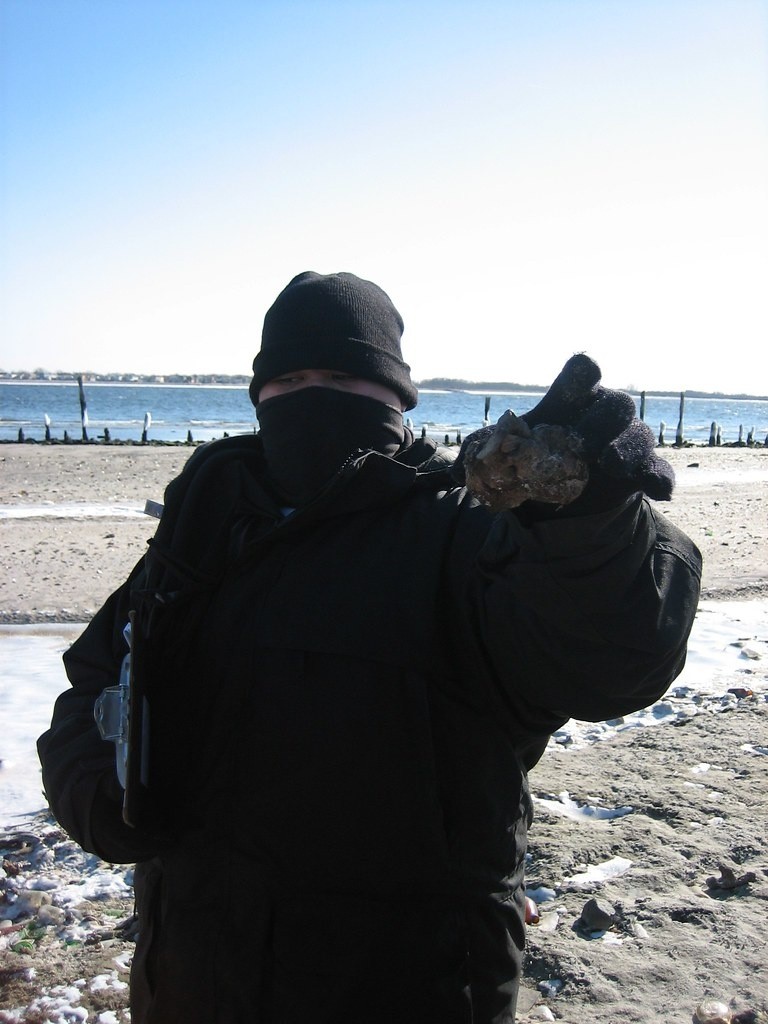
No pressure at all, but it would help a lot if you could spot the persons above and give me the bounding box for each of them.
[37,271,703,1022]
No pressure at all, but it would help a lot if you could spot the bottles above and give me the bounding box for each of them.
[526,895,540,925]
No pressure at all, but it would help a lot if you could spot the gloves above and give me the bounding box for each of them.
[89,797,156,865]
[452,353,675,522]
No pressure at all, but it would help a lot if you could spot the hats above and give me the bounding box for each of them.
[246,271,421,409]
